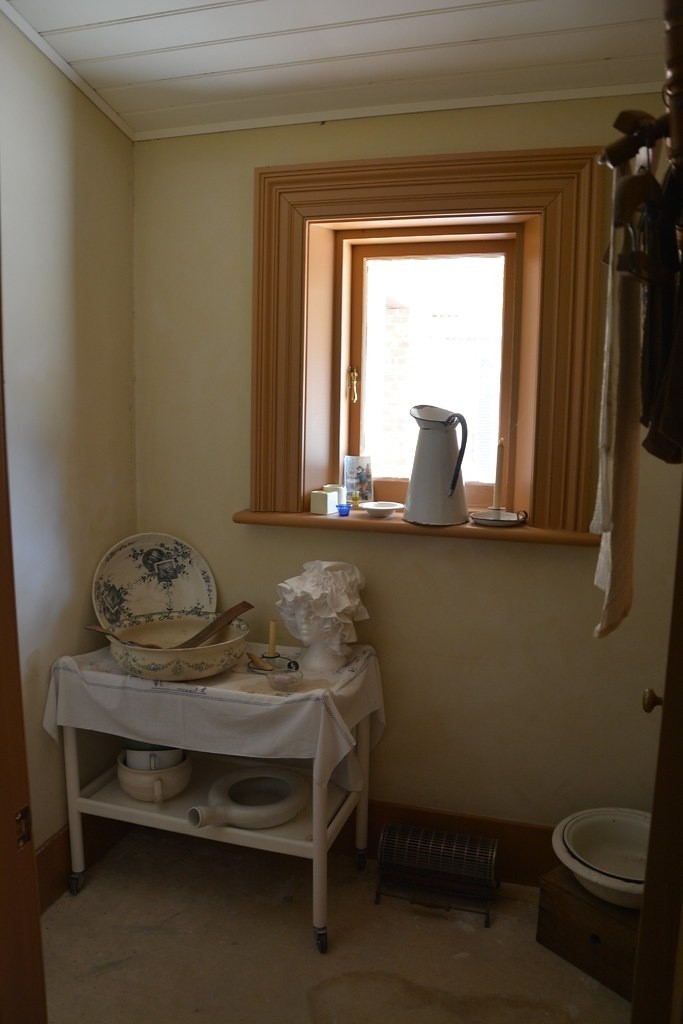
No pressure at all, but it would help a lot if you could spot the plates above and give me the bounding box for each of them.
[468,509,527,527]
[248,656,296,675]
[260,652,281,659]
[105,611,251,681]
[91,532,218,628]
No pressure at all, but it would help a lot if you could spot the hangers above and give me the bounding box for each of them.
[613,146,664,229]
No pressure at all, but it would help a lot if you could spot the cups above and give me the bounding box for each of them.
[323,483,346,509]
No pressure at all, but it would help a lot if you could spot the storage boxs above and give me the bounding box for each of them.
[537,872,637,999]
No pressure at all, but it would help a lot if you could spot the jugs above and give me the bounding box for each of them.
[401,404,471,527]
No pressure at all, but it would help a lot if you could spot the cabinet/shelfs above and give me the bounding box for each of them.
[46,645,380,953]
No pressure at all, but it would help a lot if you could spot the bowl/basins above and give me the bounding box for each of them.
[309,490,339,515]
[266,668,304,694]
[551,806,653,910]
[356,501,404,518]
[114,741,193,808]
